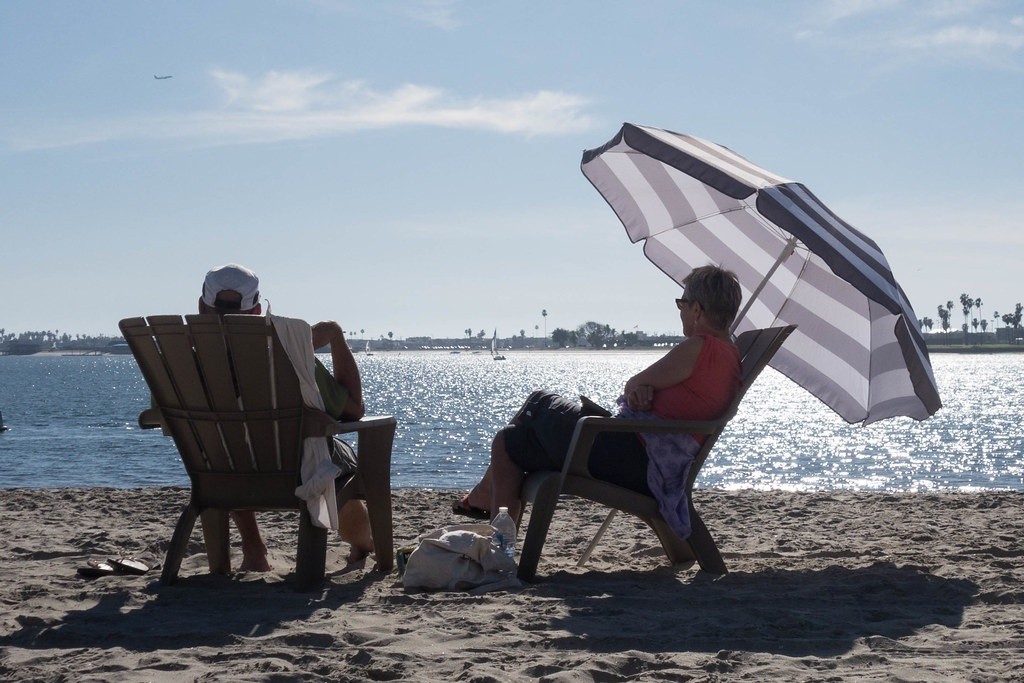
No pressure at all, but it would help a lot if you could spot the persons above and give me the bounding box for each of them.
[453,264,745,529]
[156,264,380,570]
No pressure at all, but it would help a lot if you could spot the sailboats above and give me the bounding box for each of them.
[490,329,506,360]
[365,340,373,356]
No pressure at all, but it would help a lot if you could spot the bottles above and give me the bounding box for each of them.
[491,507,516,559]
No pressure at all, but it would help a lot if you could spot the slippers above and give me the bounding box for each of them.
[453,495,491,520]
[77,561,118,573]
[108,556,149,572]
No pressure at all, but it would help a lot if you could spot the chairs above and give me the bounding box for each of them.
[504,324,799,588]
[117,313,397,593]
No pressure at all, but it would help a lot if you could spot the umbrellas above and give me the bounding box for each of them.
[576,123,941,567]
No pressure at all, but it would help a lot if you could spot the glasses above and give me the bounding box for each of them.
[676,298,705,313]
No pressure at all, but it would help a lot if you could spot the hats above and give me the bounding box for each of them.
[202,264,261,311]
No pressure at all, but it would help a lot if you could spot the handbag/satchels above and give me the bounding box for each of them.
[403,524,522,593]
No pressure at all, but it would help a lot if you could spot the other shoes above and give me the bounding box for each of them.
[397,548,414,571]
